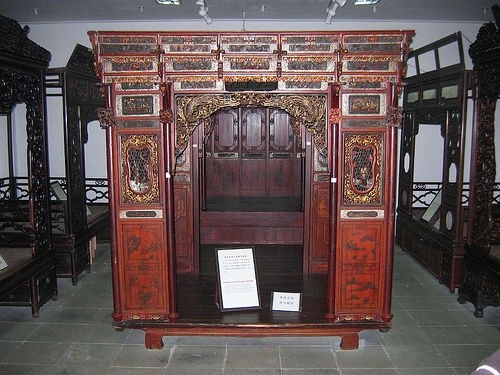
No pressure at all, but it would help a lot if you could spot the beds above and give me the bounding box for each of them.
[396,29,470,295]
[456,22,499,319]
[0,43,111,287]
[1,14,60,318]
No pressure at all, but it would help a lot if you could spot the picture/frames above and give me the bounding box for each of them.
[49,180,68,201]
[215,244,263,312]
[0,251,11,271]
[419,187,444,224]
